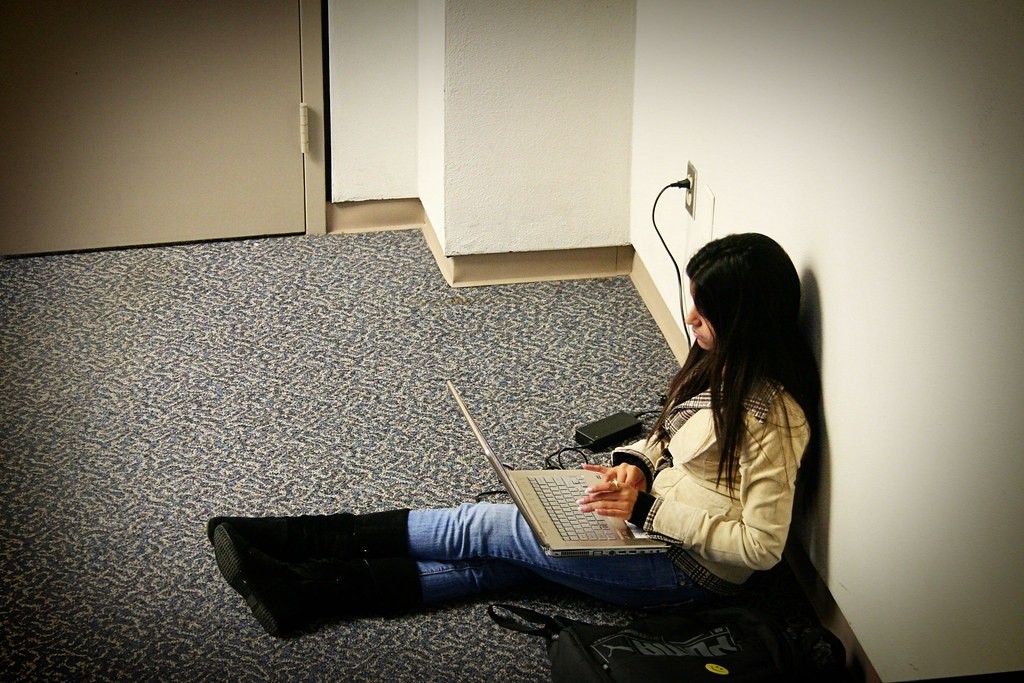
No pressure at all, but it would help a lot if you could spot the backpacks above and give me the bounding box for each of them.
[489,602,802,683]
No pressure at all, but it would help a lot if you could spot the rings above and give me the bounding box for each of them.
[613,480,621,491]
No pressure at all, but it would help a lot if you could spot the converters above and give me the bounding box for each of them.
[572,407,642,455]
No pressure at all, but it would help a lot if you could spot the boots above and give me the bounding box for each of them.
[206,508,416,564]
[213,522,426,639]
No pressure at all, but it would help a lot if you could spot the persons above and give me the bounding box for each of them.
[209,233,813,635]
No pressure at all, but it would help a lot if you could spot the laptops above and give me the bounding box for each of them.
[445,379,672,559]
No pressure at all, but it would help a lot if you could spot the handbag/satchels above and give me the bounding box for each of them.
[784,614,846,683]
